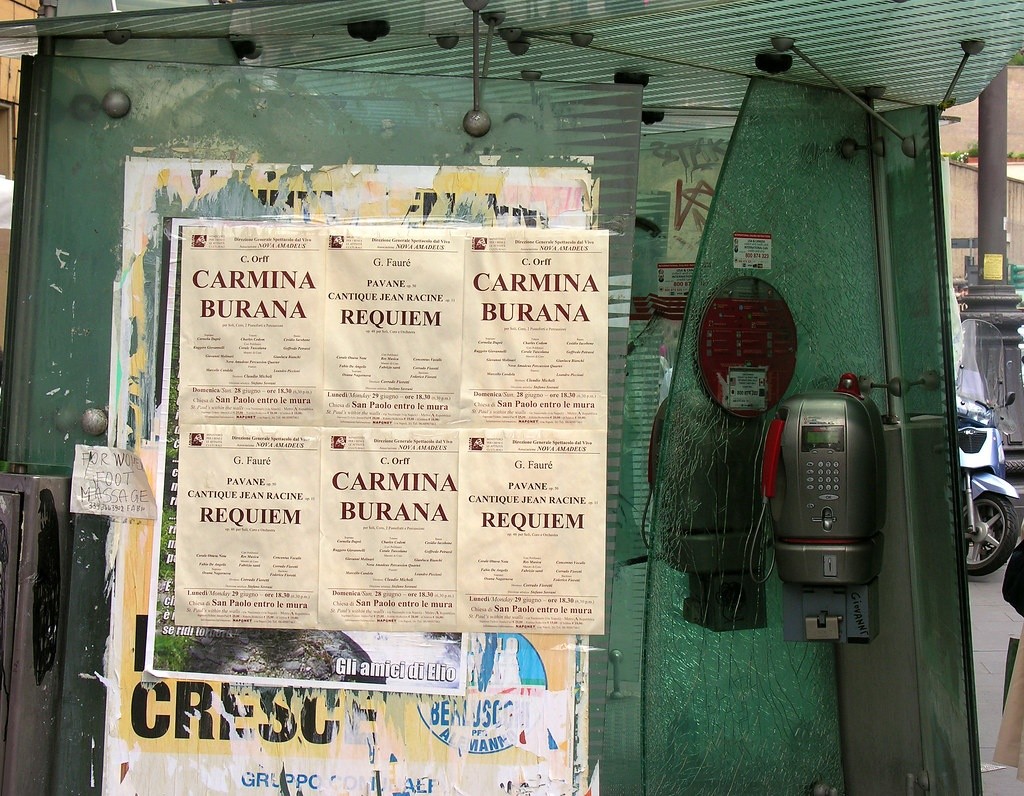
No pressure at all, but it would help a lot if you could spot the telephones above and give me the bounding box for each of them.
[644,387,765,533]
[759,390,888,541]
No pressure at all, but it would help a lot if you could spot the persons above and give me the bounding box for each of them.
[992,518,1024,783]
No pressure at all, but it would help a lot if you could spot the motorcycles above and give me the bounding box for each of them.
[952,318,1021,576]
